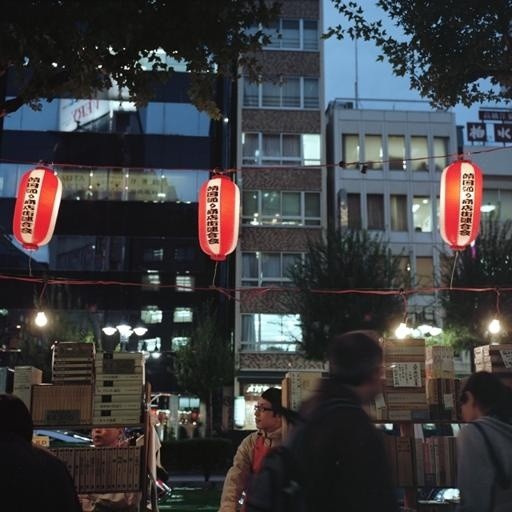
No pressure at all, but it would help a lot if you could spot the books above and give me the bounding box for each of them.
[31,335,144,491]
[1,365,43,409]
[472,342,511,384]
[370,335,461,512]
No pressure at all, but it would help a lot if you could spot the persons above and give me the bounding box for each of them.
[78,426,146,511]
[1,391,81,511]
[453,370,510,511]
[247,329,394,510]
[217,387,284,511]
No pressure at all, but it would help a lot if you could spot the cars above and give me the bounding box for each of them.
[33,430,94,449]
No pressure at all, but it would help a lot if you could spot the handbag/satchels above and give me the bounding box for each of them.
[246,446,311,512]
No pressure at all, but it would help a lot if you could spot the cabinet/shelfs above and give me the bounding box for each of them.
[280,373,511,512]
[30,381,151,512]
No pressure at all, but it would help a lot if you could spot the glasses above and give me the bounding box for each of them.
[254,405,274,412]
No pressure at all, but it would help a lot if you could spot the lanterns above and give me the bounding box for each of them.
[198,175,241,263]
[439,159,484,252]
[13,164,63,253]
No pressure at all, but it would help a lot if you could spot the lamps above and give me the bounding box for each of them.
[395,293,409,341]
[487,289,501,335]
[34,283,49,327]
